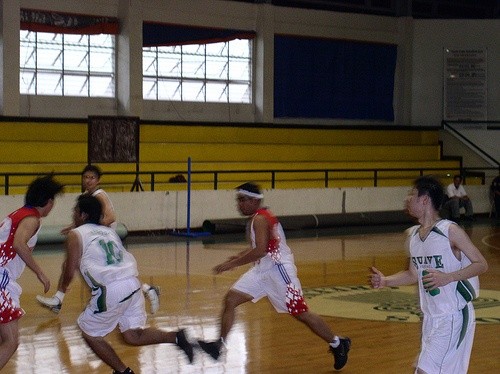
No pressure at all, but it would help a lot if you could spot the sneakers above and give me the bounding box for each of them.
[198,340,220,360]
[175,330,193,363]
[144,285,161,315]
[113,366,135,374]
[327,336,351,370]
[35,294,62,313]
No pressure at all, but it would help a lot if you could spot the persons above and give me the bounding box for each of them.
[34,164,159,316]
[367,174,490,374]
[444,174,475,222]
[62,193,193,374]
[0,171,64,372]
[196,180,351,371]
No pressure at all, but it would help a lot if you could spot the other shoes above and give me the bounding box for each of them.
[463,216,477,222]
